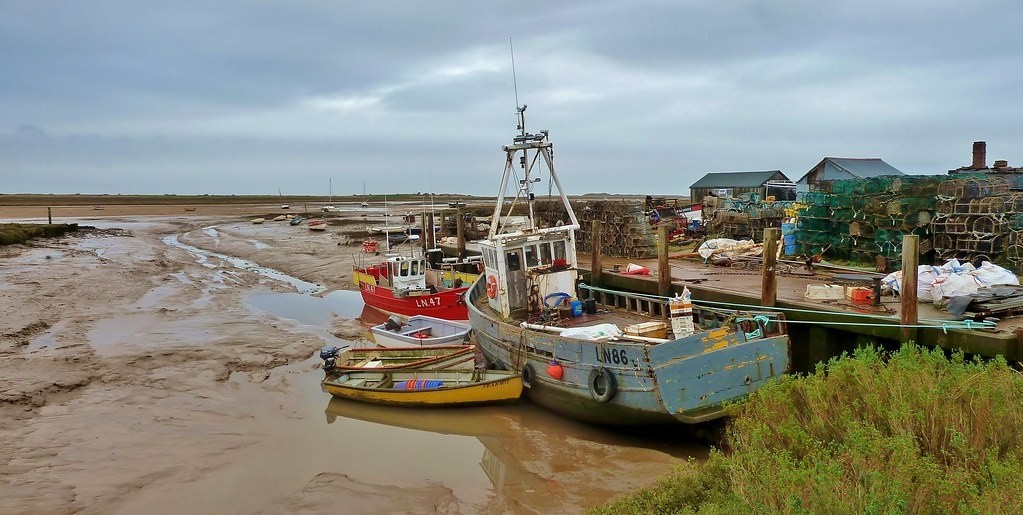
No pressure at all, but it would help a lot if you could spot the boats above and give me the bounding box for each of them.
[370,314,473,348]
[465,36,793,429]
[308,219,327,230]
[448,200,467,207]
[320,371,523,407]
[184,208,197,211]
[290,217,302,226]
[251,217,264,224]
[351,248,469,316]
[93,206,105,210]
[273,215,287,221]
[320,345,476,373]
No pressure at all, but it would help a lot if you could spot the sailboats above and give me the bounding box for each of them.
[361,181,369,207]
[320,178,340,211]
[278,186,289,209]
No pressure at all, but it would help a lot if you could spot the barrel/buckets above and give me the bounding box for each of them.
[395,380,442,389]
[782,222,796,256]
[585,298,597,314]
[571,300,582,316]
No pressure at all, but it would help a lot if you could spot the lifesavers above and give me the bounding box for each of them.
[522,365,535,390]
[588,368,617,403]
[486,275,496,299]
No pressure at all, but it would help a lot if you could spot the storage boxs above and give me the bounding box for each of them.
[365,360,381,368]
[852,288,875,304]
[846,286,867,300]
[668,297,695,339]
[624,320,666,338]
[805,283,845,299]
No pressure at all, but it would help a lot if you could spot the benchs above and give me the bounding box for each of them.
[348,378,366,388]
[401,326,432,335]
[467,370,480,385]
[370,372,393,388]
[354,355,375,367]
